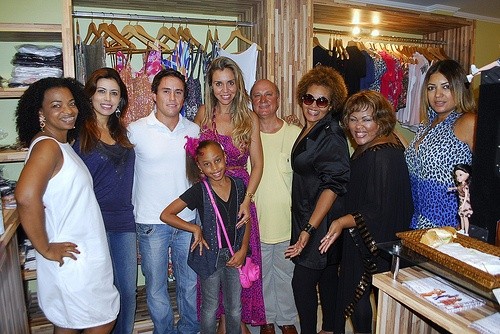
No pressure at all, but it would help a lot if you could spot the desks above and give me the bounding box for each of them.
[370,238,500,334]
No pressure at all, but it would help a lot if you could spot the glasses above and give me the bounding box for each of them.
[301,93,330,109]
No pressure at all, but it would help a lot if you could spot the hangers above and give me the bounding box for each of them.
[75,10,263,50]
[311,27,449,64]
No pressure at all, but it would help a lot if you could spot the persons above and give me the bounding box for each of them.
[289,66,346,334]
[160,140,250,334]
[67,66,135,334]
[447,164,473,236]
[249,78,304,334]
[127,68,205,334]
[14,77,120,334]
[195,57,264,334]
[406,59,478,231]
[319,90,414,334]
[420,287,461,305]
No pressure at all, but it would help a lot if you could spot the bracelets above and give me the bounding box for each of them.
[245,191,254,203]
[302,222,317,236]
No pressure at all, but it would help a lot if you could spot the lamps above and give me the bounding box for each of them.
[367,28,378,36]
[352,28,364,35]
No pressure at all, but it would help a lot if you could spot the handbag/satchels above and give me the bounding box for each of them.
[239,257,260,289]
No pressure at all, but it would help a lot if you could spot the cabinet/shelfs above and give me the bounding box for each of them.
[0,203,32,334]
[0,23,62,163]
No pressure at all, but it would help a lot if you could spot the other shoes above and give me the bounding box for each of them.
[260,323,275,334]
[278,324,298,334]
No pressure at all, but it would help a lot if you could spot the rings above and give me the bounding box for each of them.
[243,220,247,225]
[294,247,297,253]
